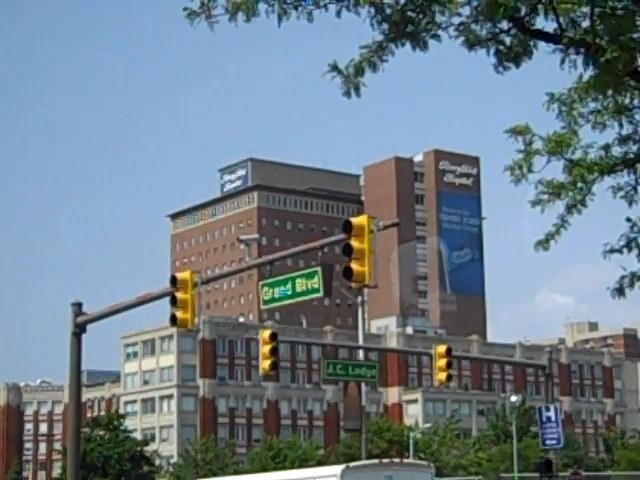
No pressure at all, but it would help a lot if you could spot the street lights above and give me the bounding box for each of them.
[507,393,526,479]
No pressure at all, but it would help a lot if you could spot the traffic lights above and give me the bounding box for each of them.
[171,270,198,329]
[343,215,374,285]
[433,344,454,385]
[258,324,278,376]
